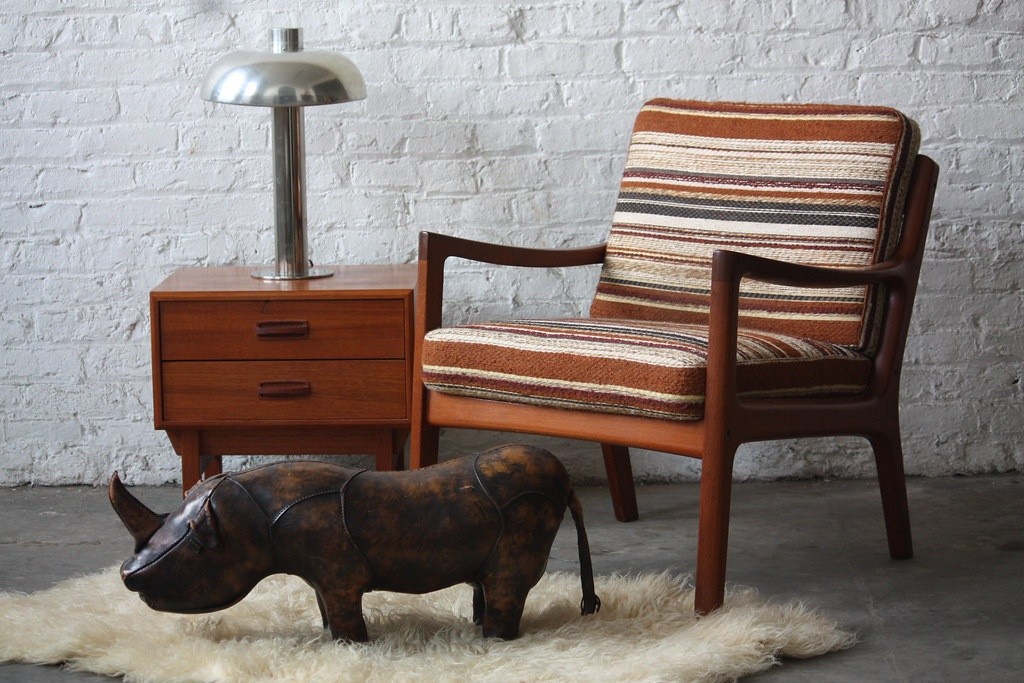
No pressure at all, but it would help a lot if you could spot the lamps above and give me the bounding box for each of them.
[200,28,368,277]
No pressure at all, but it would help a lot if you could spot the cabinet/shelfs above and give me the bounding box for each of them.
[149,263,423,499]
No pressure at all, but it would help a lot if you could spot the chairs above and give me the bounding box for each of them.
[409,95,942,623]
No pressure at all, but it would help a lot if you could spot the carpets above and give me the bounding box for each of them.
[0,556,864,683]
